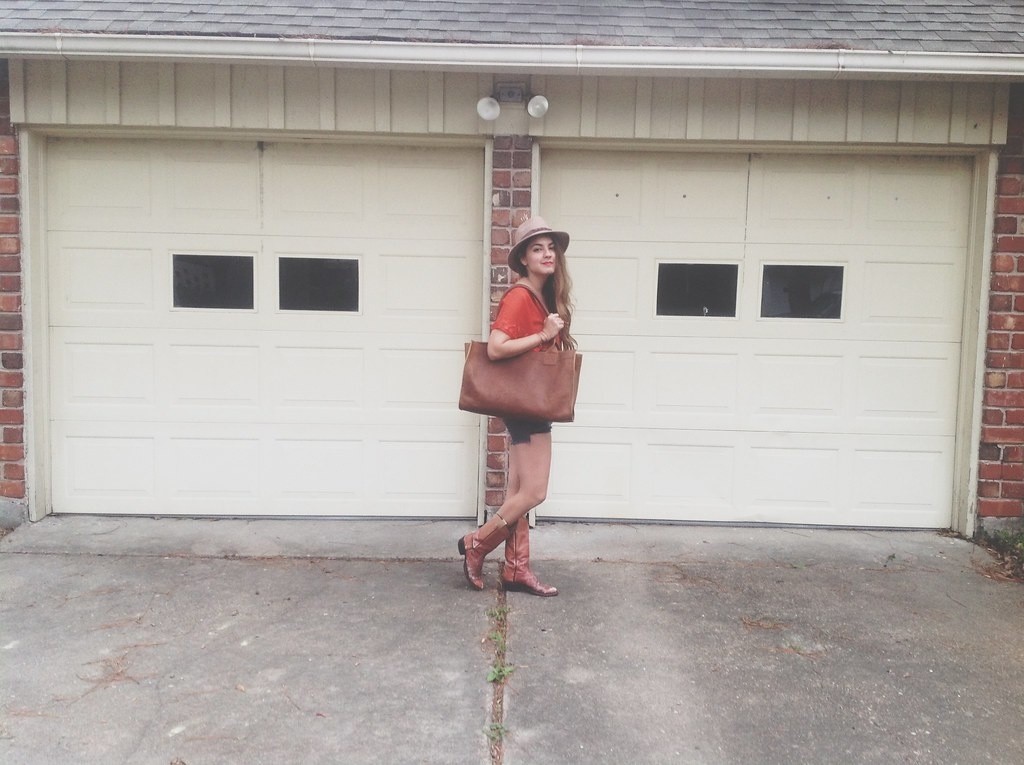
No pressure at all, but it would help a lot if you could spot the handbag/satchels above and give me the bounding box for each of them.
[458,285,582,422]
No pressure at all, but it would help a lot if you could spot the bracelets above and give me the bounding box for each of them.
[537,330,549,345]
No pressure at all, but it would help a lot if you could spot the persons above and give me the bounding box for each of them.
[458,217,579,597]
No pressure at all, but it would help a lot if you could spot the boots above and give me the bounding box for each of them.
[503,517,559,596]
[458,514,512,591]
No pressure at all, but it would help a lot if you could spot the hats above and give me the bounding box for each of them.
[508,217,570,278]
